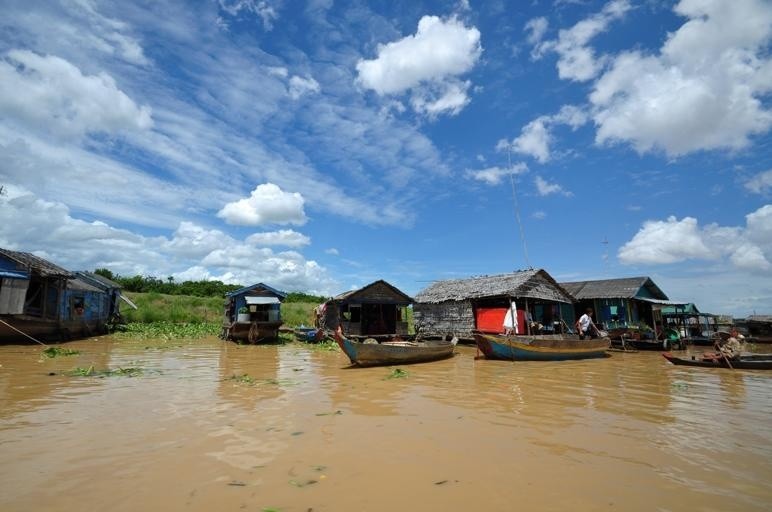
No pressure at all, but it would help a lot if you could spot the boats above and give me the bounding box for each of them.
[662,352,772,369]
[335,330,456,368]
[221,282,289,341]
[2,249,130,347]
[473,332,613,357]
[293,280,413,346]
[627,338,676,350]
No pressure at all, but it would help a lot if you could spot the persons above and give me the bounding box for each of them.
[577,307,602,341]
[727,326,745,345]
[703,331,741,366]
